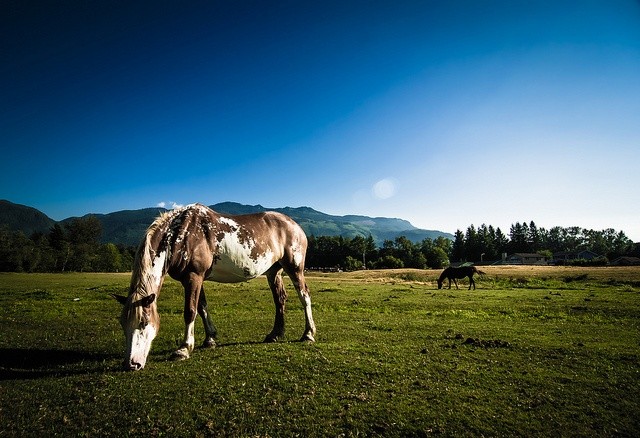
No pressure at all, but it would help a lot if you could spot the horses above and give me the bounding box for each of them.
[115,202,317,372]
[436,266,486,290]
